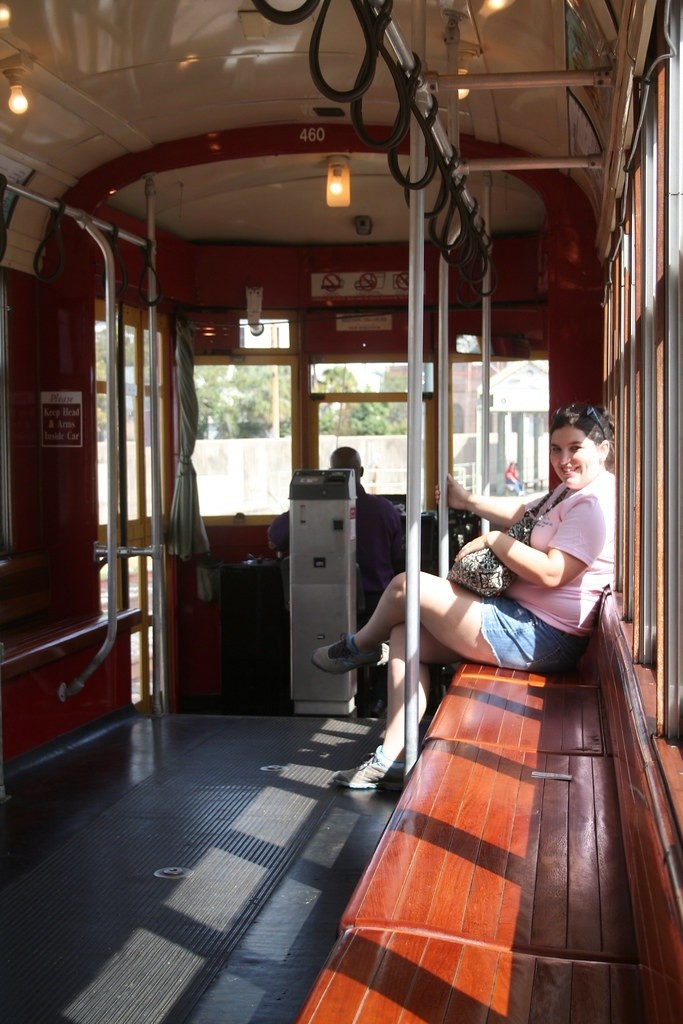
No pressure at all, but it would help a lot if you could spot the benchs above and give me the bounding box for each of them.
[0,550,146,767]
[294,584,682,1024]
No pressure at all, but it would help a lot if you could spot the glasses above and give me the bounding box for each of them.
[554,405,604,430]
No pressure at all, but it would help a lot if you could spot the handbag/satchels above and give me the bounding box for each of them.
[446,487,569,598]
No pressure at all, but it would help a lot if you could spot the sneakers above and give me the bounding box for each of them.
[310,632,382,673]
[332,744,421,791]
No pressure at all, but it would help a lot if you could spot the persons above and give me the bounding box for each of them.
[505,463,527,496]
[266,448,402,719]
[311,399,615,790]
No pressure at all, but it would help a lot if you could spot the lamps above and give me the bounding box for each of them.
[325,156,351,208]
